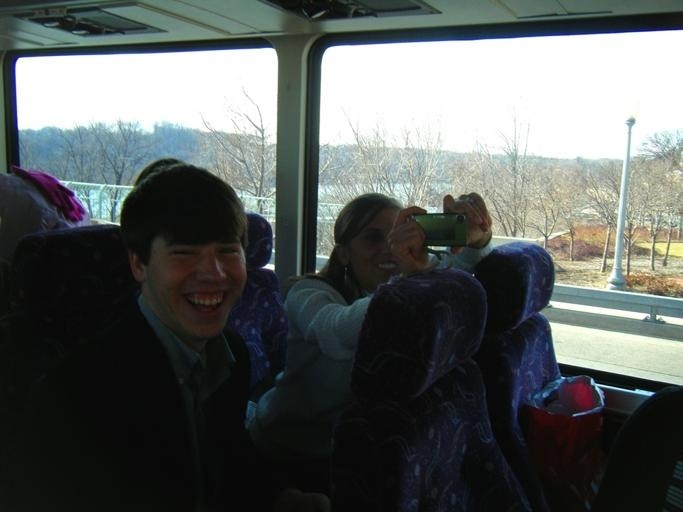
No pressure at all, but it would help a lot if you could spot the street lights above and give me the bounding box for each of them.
[603,118,635,291]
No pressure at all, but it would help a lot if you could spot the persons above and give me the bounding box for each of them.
[240,194,494,490]
[17,159,333,509]
[135,157,183,188]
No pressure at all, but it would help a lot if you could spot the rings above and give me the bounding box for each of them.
[464,196,473,204]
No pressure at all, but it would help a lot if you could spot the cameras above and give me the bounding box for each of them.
[409,212,467,247]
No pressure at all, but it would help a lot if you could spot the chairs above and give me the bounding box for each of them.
[0,207,566,512]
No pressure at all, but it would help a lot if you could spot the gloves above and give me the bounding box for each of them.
[8,163,85,222]
